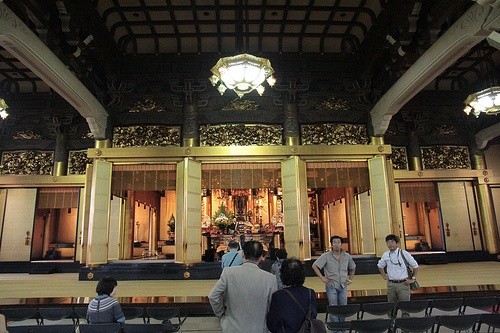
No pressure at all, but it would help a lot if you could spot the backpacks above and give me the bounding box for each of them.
[280,290,327,333]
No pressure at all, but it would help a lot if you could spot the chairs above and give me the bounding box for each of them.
[1,307,189,333]
[325,296,500,333]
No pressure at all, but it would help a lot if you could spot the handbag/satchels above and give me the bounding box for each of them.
[401,250,419,291]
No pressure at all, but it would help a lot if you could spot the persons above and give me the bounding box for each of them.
[222,241,244,269]
[231,217,236,230]
[258,248,279,273]
[209,241,278,333]
[377,234,419,333]
[86,276,125,328]
[312,236,356,333]
[238,235,245,251]
[271,249,290,289]
[267,259,317,333]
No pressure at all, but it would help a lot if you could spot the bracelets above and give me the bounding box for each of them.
[347,279,351,283]
[411,276,416,279]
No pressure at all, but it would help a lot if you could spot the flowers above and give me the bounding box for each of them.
[213,203,234,235]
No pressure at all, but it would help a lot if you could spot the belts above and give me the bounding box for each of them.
[389,278,408,283]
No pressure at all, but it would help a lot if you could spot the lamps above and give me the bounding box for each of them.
[209,0,276,99]
[462,47,500,119]
